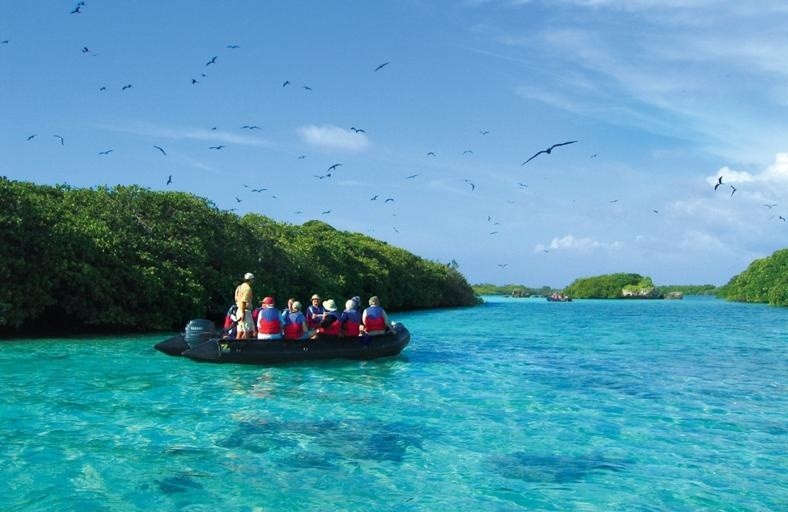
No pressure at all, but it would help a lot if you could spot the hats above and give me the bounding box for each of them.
[322,299,338,312]
[368,296,380,305]
[310,293,322,303]
[243,273,255,280]
[351,296,359,305]
[264,297,274,304]
[258,297,266,303]
[344,300,354,309]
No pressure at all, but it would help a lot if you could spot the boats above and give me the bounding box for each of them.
[547,296,573,302]
[505,288,533,299]
[152,320,411,366]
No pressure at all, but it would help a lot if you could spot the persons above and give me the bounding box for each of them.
[223,272,397,341]
[551,293,568,302]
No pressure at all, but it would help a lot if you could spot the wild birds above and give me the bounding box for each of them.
[715,176,723,190]
[190,57,216,88]
[347,124,366,134]
[372,60,390,73]
[56,136,64,144]
[371,193,395,205]
[298,154,309,161]
[478,129,490,138]
[152,143,174,187]
[280,78,313,96]
[462,148,475,154]
[425,151,434,158]
[315,162,344,184]
[97,147,116,158]
[488,213,500,234]
[227,46,239,48]
[406,173,419,179]
[391,214,401,235]
[610,200,618,203]
[97,83,133,93]
[206,123,224,153]
[294,210,333,215]
[239,123,261,132]
[731,186,736,196]
[227,184,278,212]
[464,179,477,192]
[82,47,88,53]
[764,204,776,208]
[780,216,785,221]
[520,140,578,167]
[498,264,508,267]
[27,135,37,141]
[515,180,529,189]
[71,6,80,14]
